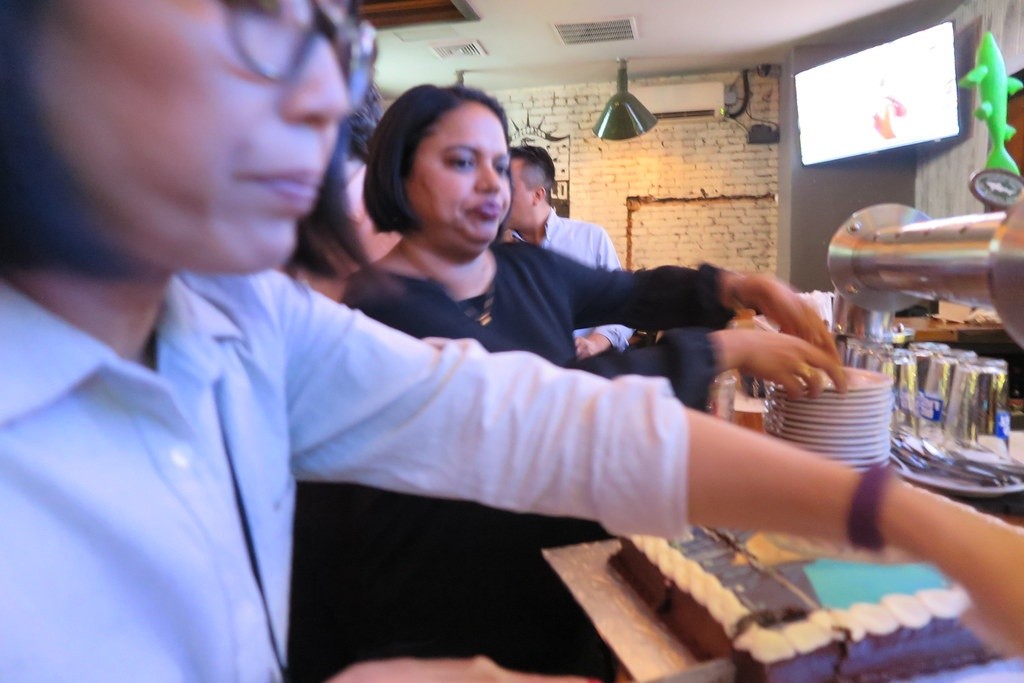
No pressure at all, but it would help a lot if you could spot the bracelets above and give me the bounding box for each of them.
[845,465,898,553]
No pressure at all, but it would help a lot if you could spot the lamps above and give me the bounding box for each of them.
[593,57,658,140]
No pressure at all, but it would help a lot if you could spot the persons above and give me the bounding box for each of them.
[290,85,636,359]
[0,0,1024,683]
[284,85,851,683]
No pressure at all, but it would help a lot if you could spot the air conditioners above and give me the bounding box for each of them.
[609,82,725,124]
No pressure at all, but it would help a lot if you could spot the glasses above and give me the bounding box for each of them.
[225,0,380,107]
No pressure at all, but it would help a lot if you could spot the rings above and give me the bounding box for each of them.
[799,364,812,378]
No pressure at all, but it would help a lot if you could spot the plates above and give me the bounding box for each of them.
[762,366,895,474]
[892,450,1024,498]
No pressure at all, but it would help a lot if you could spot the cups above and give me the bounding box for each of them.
[843,336,1010,453]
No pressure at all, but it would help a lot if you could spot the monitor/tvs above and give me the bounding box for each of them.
[794,20,965,166]
